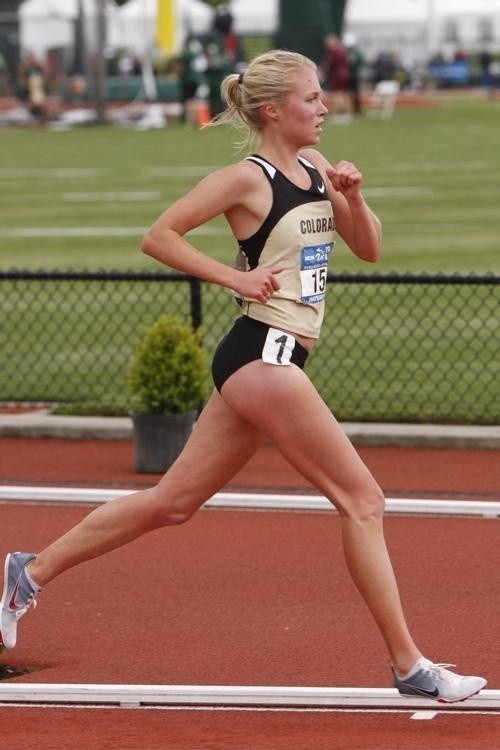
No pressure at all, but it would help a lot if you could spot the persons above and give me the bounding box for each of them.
[0,51,489,703]
[319,32,401,121]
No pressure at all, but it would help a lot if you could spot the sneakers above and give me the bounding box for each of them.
[0,553,40,650]
[390,664,487,703]
